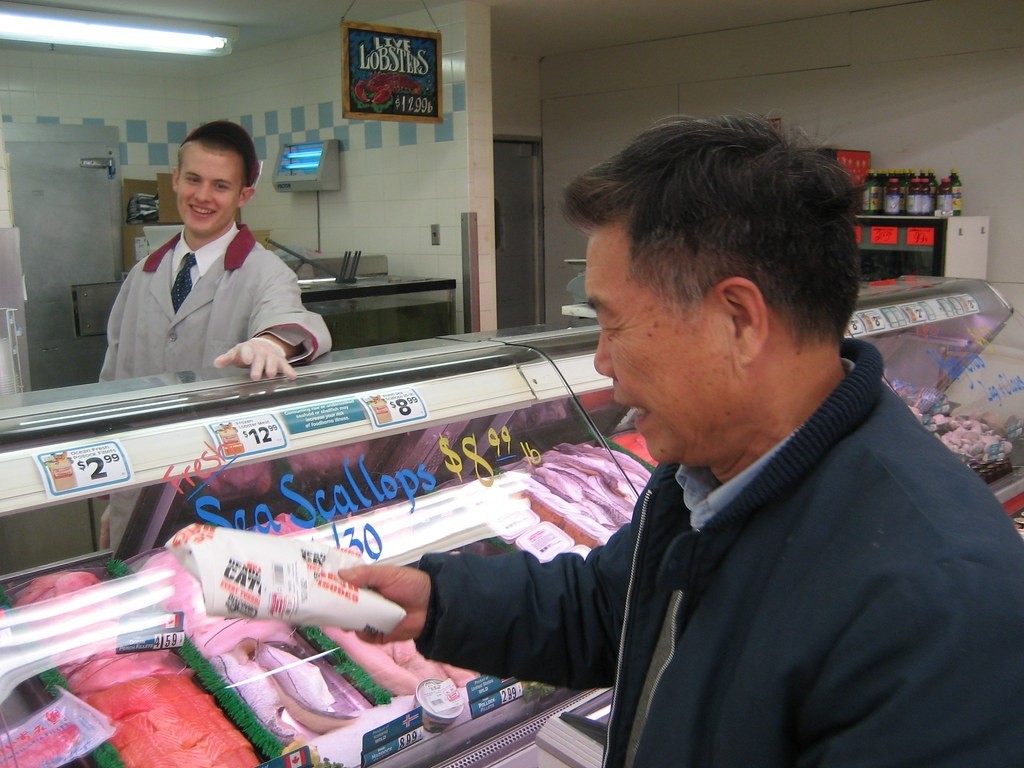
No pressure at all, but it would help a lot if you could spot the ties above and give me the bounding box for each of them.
[171,252,197,315]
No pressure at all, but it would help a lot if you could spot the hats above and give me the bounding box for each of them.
[180,121,258,188]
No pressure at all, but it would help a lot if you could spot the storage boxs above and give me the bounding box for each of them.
[122,173,271,276]
[817,148,872,187]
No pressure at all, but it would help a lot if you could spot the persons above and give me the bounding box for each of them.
[338,114,1024,768]
[99,120,332,559]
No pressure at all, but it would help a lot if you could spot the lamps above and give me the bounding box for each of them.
[0,1,241,57]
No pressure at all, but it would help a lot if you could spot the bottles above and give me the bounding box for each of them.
[861,168,963,216]
